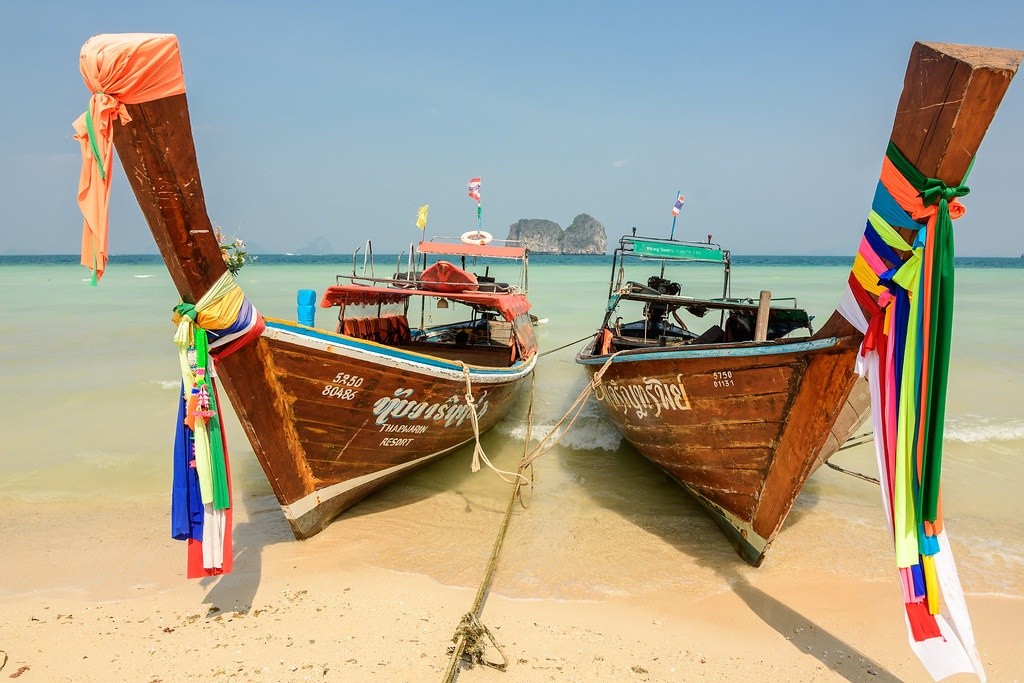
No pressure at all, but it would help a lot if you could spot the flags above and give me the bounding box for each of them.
[466,175,481,201]
[671,193,685,217]
[416,205,430,230]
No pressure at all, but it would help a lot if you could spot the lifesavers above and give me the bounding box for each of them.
[460,230,494,246]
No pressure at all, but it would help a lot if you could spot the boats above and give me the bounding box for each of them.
[78,31,540,541]
[573,38,1023,568]
